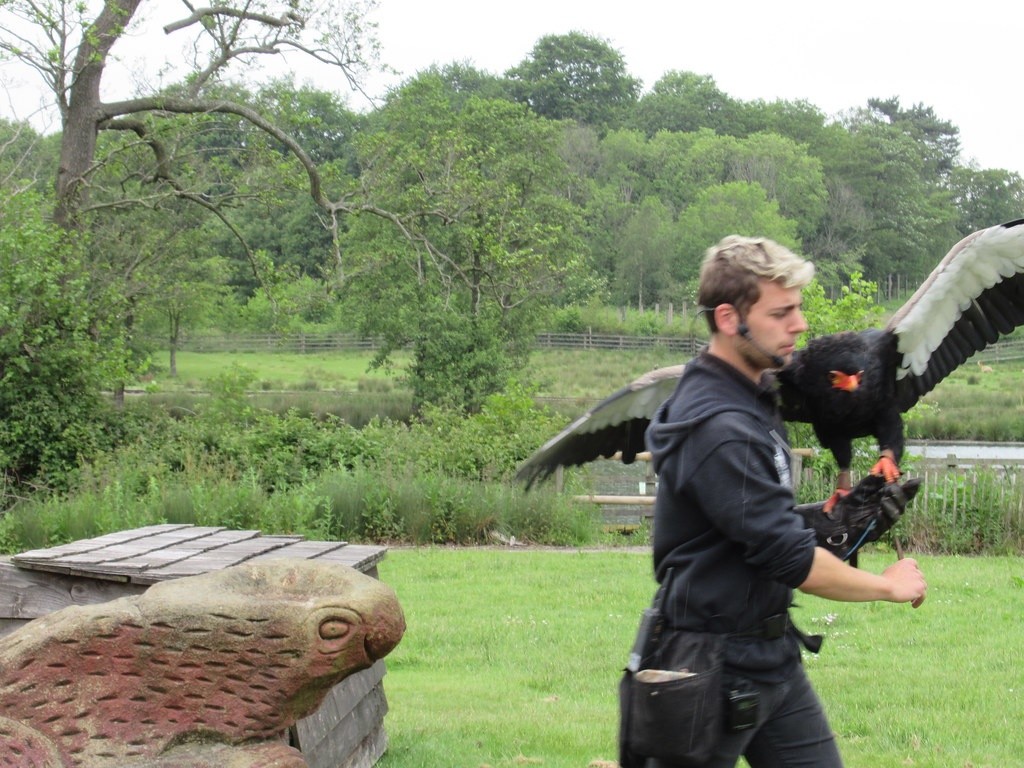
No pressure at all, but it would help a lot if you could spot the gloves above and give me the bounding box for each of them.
[792,471,922,545]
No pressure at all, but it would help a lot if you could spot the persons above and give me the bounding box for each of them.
[615,233,927,768]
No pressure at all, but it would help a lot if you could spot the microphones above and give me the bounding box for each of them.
[744,334,785,368]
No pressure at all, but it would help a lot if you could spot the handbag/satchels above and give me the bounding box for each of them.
[615,630,722,766]
